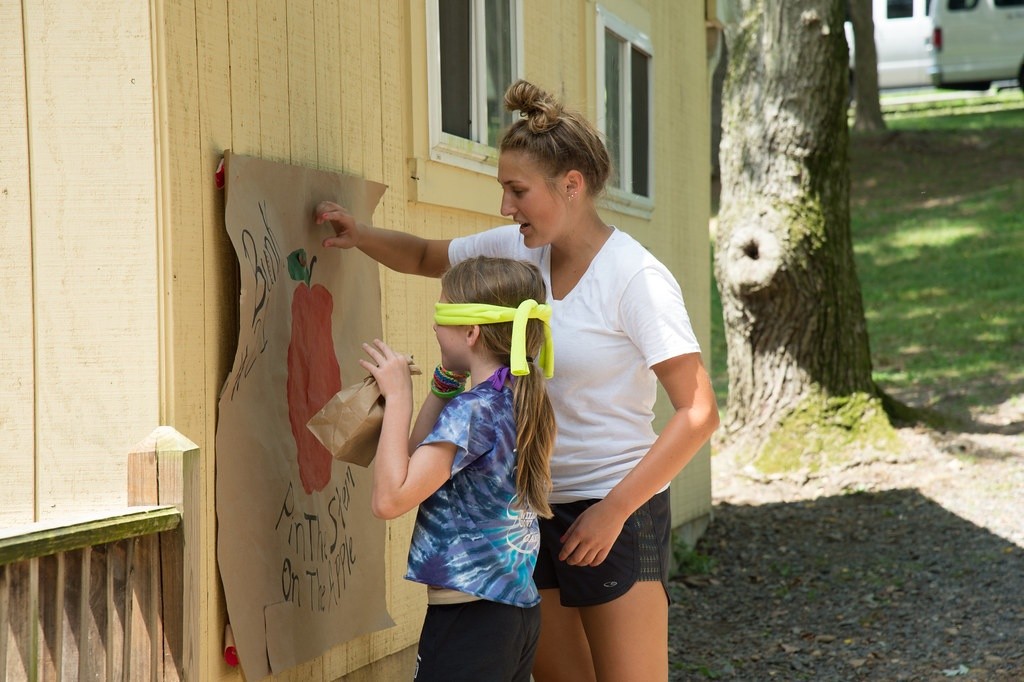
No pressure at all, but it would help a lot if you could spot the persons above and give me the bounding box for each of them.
[356,252,555,682]
[311,78,723,682]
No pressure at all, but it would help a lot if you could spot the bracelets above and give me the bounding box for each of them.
[429,361,472,401]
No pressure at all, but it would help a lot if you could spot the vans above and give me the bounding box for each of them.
[925,0,1023,98]
[844,0,933,99]
